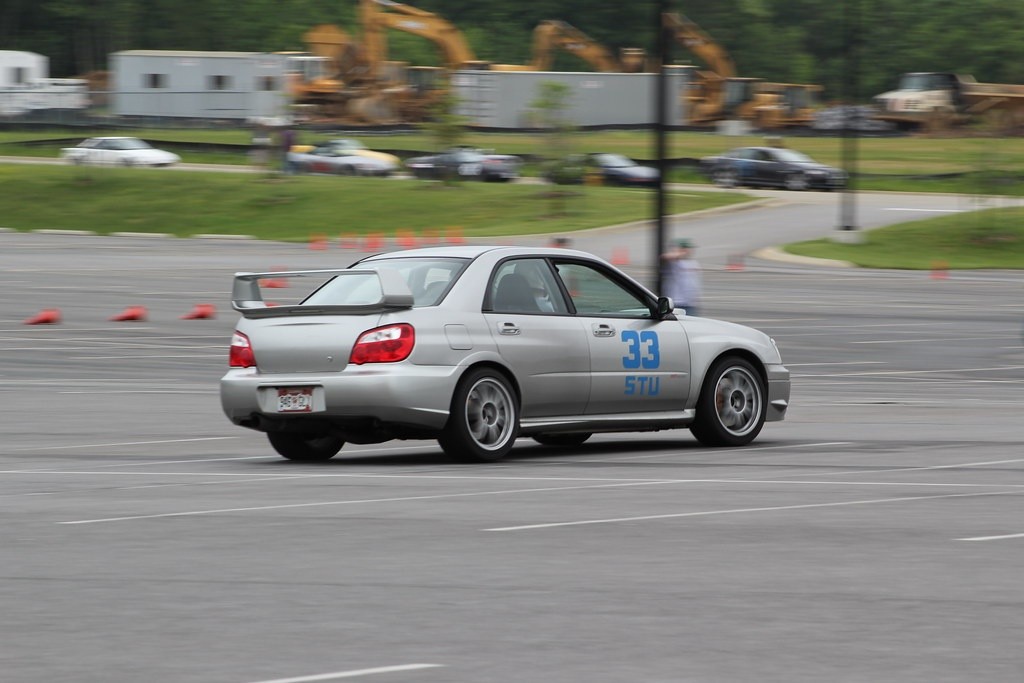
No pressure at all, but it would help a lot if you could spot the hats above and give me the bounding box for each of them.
[679,238,696,249]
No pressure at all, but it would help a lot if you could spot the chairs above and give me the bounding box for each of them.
[427,281,449,302]
[494,274,540,312]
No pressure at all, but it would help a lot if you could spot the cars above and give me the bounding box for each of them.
[700,146,847,195]
[409,146,525,184]
[545,153,660,190]
[217,245,792,462]
[59,136,183,168]
[286,137,400,175]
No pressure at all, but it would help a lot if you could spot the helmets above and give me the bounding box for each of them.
[514,262,547,290]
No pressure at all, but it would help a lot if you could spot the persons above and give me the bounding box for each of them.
[513,263,555,314]
[246,119,300,178]
[660,237,703,317]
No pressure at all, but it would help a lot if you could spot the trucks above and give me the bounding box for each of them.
[869,76,1021,130]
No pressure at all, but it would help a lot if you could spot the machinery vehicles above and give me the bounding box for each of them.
[284,0,825,145]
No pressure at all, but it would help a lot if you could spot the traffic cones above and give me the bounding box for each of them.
[19,275,291,324]
[308,223,630,298]
